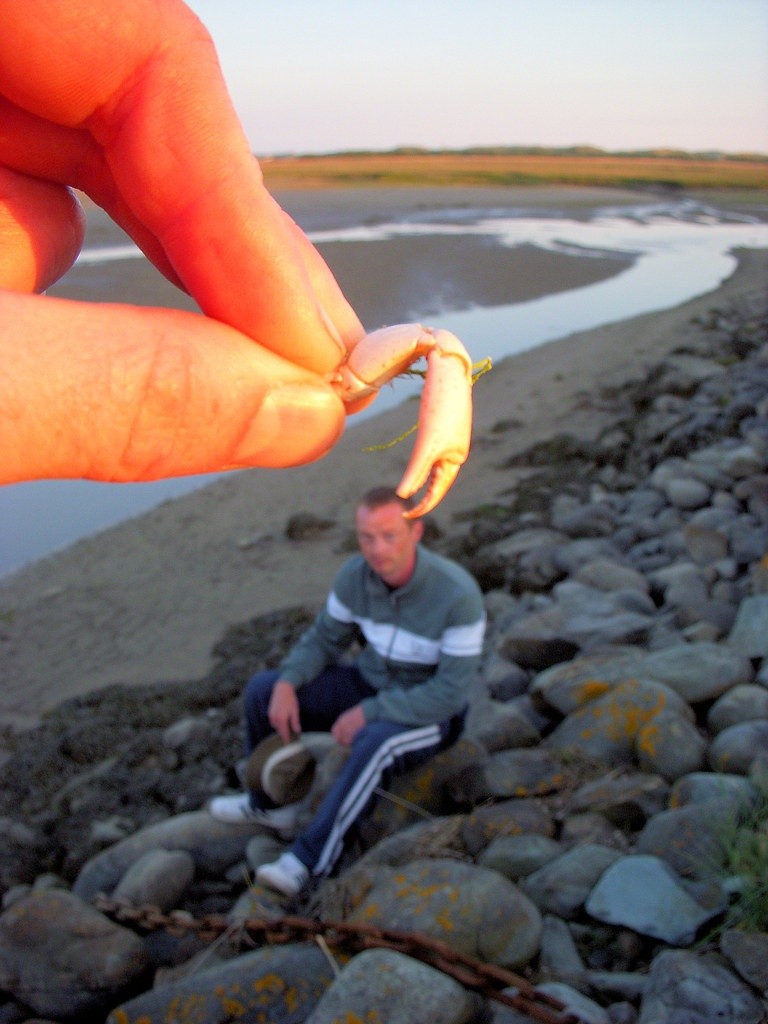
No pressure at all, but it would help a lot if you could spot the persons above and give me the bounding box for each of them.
[208,485,485,900]
[0,0,368,482]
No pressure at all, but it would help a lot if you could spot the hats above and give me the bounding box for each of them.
[246,731,350,810]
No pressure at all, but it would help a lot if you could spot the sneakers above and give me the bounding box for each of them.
[255,852,311,897]
[209,795,296,829]
